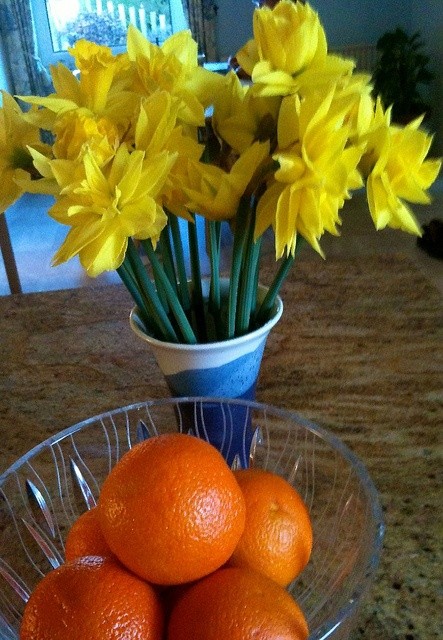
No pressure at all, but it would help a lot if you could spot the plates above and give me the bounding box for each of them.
[0,395,388,640]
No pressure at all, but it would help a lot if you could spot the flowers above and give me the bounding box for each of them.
[0,2,443,344]
